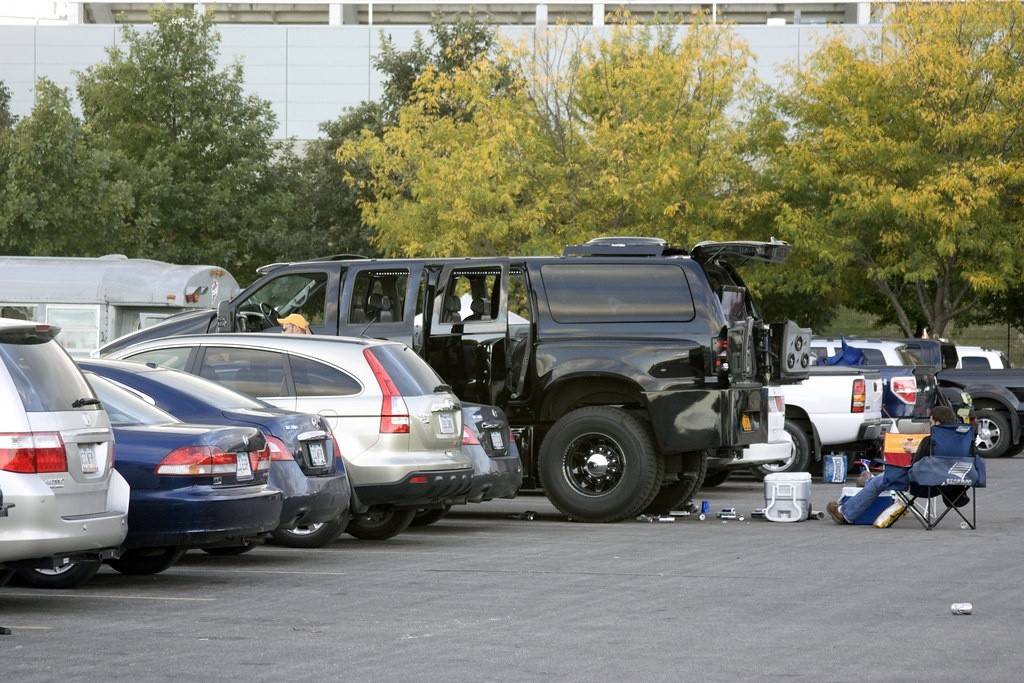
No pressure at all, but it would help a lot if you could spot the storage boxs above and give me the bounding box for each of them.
[840,487,894,525]
[823,455,847,483]
[764,471,813,522]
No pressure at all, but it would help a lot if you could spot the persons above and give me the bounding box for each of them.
[277,313,309,397]
[826,405,956,525]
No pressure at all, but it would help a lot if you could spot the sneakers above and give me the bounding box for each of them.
[826,502,844,524]
[855,470,872,487]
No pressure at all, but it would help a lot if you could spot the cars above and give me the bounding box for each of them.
[0,317,524,595]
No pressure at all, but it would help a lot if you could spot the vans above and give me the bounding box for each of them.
[697,335,1024,481]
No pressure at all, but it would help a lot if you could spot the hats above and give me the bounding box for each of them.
[277,314,309,330]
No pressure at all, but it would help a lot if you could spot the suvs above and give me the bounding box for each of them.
[94,235,790,523]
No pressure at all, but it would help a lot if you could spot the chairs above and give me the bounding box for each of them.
[464,297,492,322]
[444,295,461,323]
[874,424,987,531]
[368,294,396,321]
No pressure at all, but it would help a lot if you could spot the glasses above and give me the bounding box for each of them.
[282,326,296,331]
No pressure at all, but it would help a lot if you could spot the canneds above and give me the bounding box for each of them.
[636,500,765,522]
[568,517,573,521]
[507,511,538,520]
[950,603,972,614]
[960,522,969,529]
